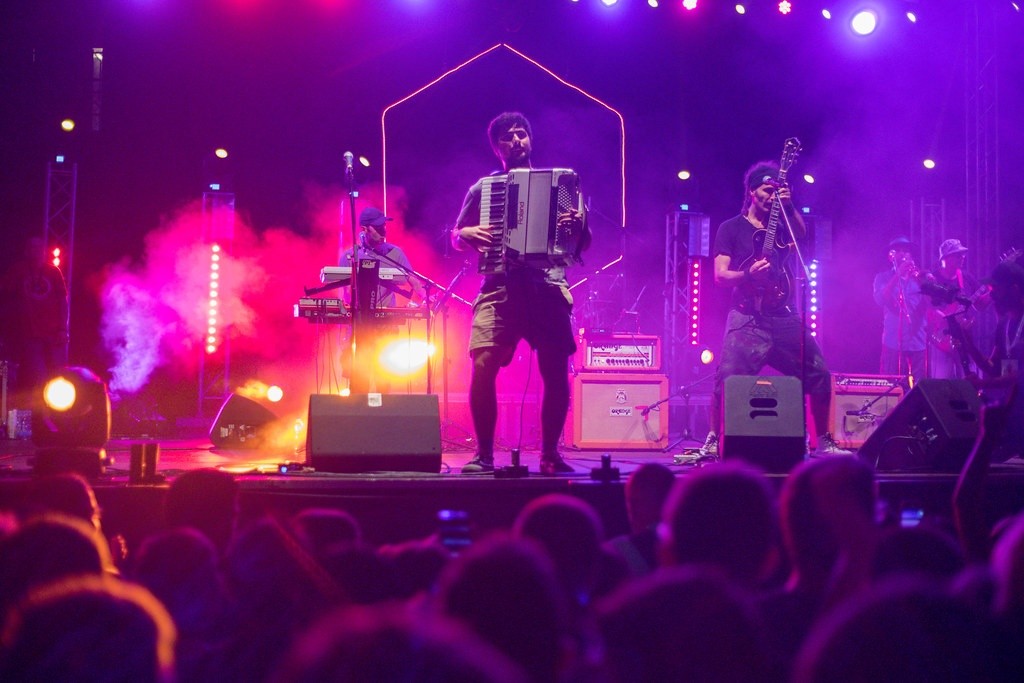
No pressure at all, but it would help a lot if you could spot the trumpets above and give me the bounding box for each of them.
[901,257,921,282]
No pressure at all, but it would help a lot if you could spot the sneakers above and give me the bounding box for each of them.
[700,431,718,454]
[809,432,853,458]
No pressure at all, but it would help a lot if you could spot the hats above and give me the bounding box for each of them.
[939,239,968,262]
[889,236,911,249]
[977,262,1024,284]
[359,208,393,225]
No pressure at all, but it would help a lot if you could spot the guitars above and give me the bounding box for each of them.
[732,136,803,313]
[926,244,1023,354]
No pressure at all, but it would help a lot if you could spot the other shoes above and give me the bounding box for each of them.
[541,449,576,472]
[460,453,494,471]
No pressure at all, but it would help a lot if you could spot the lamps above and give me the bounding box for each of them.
[27,366,112,470]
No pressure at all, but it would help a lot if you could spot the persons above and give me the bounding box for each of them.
[451,110,594,476]
[875,238,930,383]
[949,260,1024,462]
[925,238,992,381]
[699,160,855,459]
[339,208,421,395]
[0,385,1024,683]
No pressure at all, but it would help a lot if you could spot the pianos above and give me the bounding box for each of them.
[293,265,432,395]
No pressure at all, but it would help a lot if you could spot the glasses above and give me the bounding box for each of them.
[948,253,966,259]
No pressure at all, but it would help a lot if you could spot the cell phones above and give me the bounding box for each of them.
[437,512,475,555]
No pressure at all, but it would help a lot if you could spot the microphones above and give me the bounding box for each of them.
[641,408,650,416]
[763,176,787,188]
[358,231,367,255]
[343,151,353,170]
[846,410,871,415]
[890,249,896,259]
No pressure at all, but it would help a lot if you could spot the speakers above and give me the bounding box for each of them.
[718,374,807,474]
[823,372,991,474]
[306,393,442,474]
[210,392,281,451]
[564,372,669,451]
